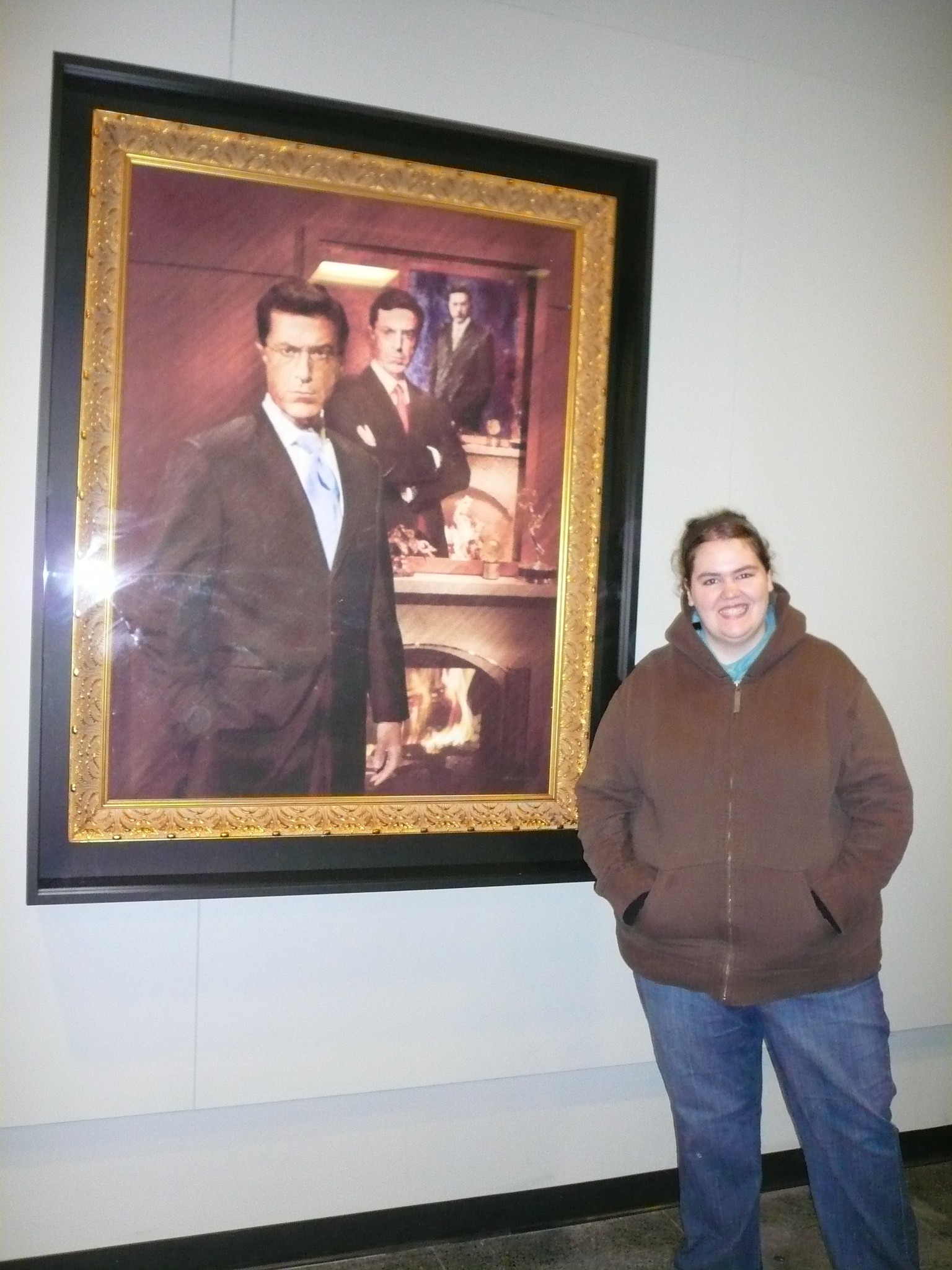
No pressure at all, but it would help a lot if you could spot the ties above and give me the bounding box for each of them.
[393,384,409,437]
[294,433,343,571]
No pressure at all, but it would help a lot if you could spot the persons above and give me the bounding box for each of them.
[576,511,920,1270]
[138,282,493,797]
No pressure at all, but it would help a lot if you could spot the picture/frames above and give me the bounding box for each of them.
[26,51,661,909]
